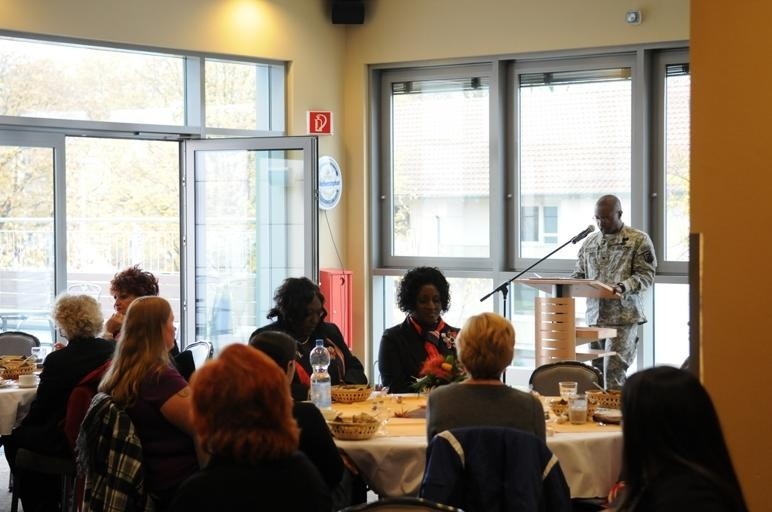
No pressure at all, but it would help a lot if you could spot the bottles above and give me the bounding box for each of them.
[310,339,331,408]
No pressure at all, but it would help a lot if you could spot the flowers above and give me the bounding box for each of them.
[408,354,469,400]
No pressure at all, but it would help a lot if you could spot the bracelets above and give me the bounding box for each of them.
[102,332,113,337]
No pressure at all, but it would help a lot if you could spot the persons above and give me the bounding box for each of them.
[426,312,546,447]
[173,342,331,512]
[103,268,179,359]
[379,267,467,394]
[250,331,345,491]
[604,365,749,512]
[570,194,657,392]
[249,277,371,402]
[3,294,117,512]
[97,295,196,511]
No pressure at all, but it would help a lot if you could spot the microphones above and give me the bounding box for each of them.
[574,225,596,245]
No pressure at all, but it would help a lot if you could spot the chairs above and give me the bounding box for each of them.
[73,392,147,512]
[337,496,465,512]
[419,425,570,512]
[182,341,214,369]
[11,417,68,511]
[529,361,604,396]
[0,332,40,357]
[174,350,194,382]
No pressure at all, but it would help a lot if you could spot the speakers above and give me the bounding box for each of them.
[329,0,367,25]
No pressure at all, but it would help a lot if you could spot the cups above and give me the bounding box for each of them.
[559,382,577,401]
[31,347,46,364]
[19,375,36,387]
[569,400,587,425]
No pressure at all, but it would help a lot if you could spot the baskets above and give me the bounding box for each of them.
[550,401,597,417]
[327,420,379,440]
[586,390,622,409]
[0,360,36,380]
[331,385,372,403]
